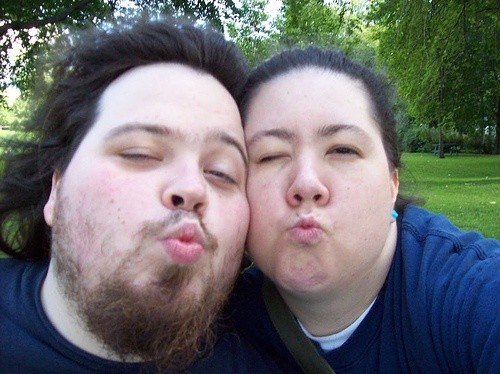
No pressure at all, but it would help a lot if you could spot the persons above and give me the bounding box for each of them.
[236,47,500,374]
[0,18,285,374]
